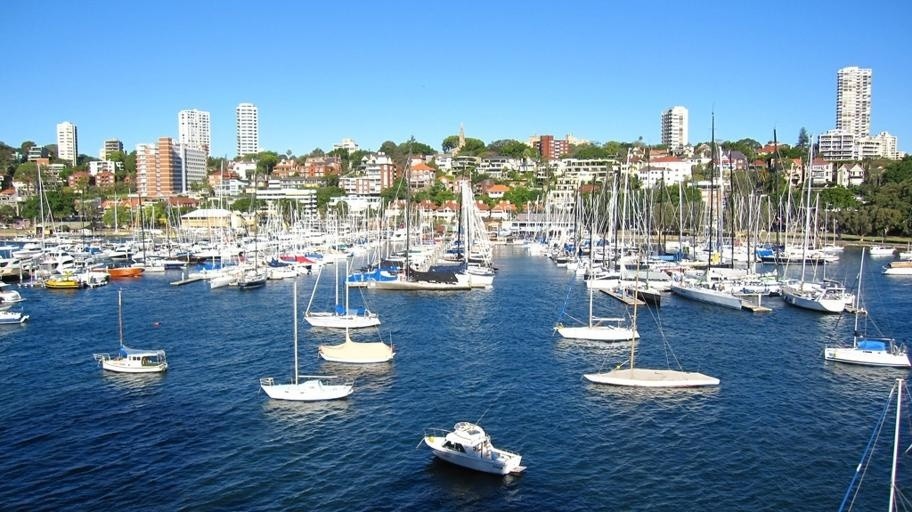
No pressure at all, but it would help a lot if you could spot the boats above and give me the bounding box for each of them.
[92,291,168,373]
[424,422,527,476]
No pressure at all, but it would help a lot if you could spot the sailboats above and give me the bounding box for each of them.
[824,246,911,370]
[260,282,355,402]
[319,262,393,364]
[582,247,720,388]
